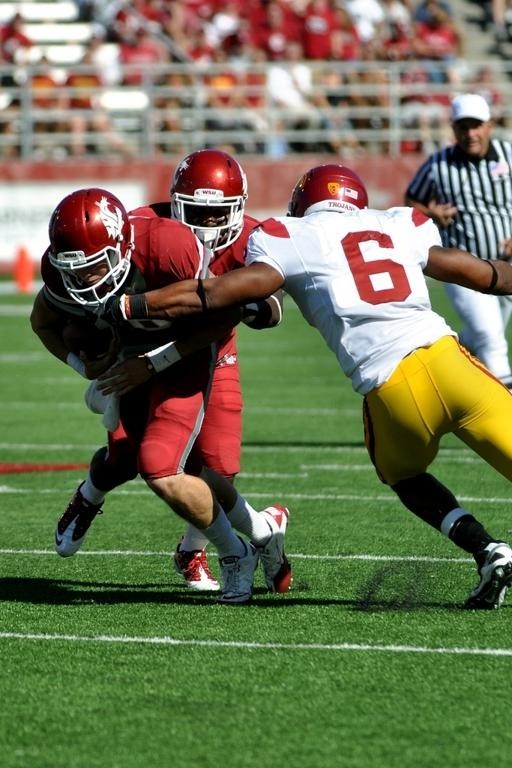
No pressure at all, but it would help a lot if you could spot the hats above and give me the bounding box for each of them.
[449,94,491,126]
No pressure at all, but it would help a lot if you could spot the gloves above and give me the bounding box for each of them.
[101,293,129,329]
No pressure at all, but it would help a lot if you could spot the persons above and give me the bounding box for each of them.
[54,149,284,558]
[29,186,292,602]
[1,0,512,158]
[405,93,512,388]
[100,161,512,611]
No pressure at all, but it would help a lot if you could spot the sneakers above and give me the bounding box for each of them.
[217,536,259,602]
[172,543,221,593]
[254,503,293,594]
[54,480,106,559]
[460,539,512,610]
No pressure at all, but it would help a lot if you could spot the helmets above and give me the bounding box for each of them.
[169,149,248,204]
[47,187,132,264]
[287,163,368,217]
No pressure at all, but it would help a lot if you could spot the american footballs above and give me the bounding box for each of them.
[62,319,111,361]
